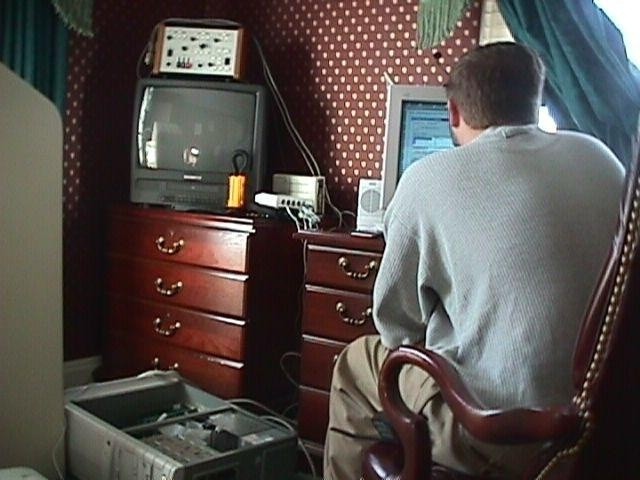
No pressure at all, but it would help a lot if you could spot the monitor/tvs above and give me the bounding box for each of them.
[382,85,458,210]
[124,77,266,213]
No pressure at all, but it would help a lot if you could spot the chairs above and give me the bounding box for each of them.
[360,139,640,479]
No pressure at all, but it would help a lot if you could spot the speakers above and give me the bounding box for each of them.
[356,178,384,235]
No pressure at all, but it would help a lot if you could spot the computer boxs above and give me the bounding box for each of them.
[63,371,296,479]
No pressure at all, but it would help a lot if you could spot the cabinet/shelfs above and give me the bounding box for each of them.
[102,205,300,420]
[298,234,384,453]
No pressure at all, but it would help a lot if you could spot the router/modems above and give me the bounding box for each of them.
[254,190,313,212]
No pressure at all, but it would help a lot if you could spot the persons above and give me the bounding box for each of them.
[323,41,628,480]
